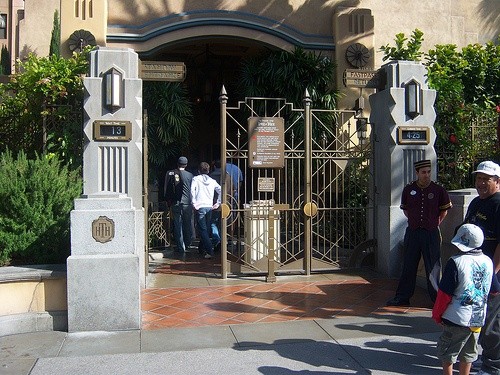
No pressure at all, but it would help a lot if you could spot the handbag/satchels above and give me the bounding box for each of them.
[165,169,183,206]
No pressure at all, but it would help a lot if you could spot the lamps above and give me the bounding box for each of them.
[102,66,124,115]
[405,78,422,121]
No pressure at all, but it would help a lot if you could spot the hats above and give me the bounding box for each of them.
[471,161,500,177]
[414,160,431,168]
[179,157,187,164]
[451,224,484,252]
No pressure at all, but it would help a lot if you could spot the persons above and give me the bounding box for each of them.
[189,162,222,261]
[207,160,235,252]
[218,162,243,246]
[164,156,194,254]
[385,159,453,306]
[459,160,500,375]
[432,223,500,375]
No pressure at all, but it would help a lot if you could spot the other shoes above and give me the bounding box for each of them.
[387,297,409,306]
[204,254,214,259]
